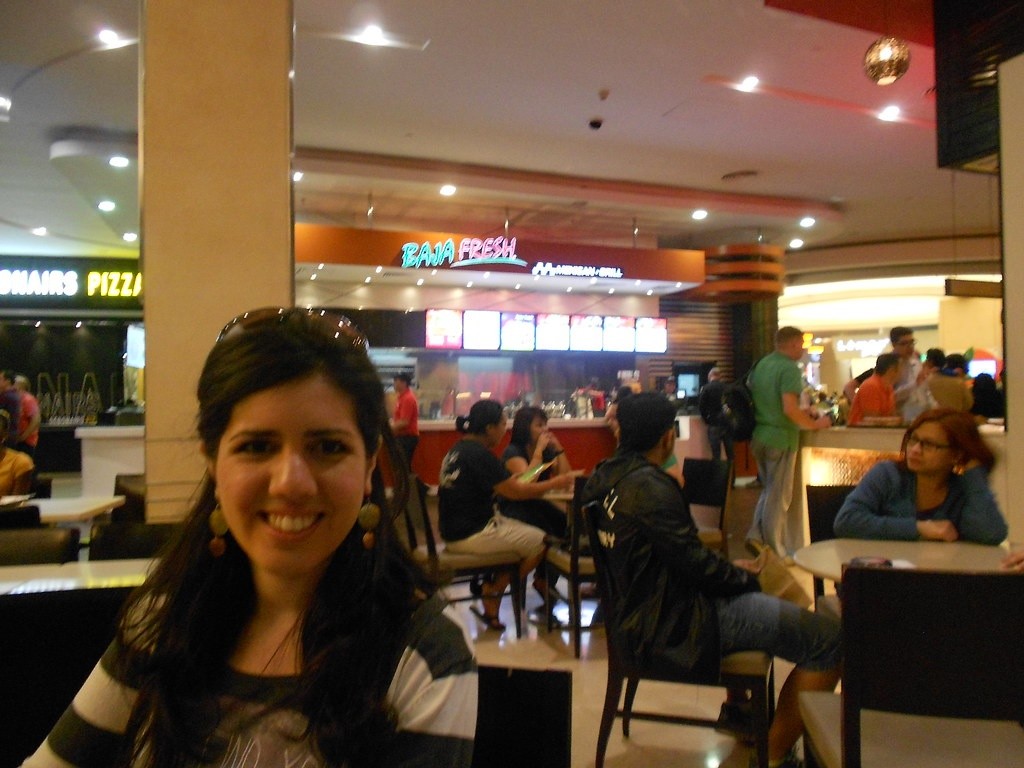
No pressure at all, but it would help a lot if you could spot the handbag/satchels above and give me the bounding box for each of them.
[721,382,756,441]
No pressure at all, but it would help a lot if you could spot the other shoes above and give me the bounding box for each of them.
[748,748,804,768]
[715,698,758,734]
[744,537,765,558]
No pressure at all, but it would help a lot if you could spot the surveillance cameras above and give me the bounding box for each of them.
[589,120,602,129]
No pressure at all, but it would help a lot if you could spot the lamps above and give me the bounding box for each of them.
[864,0,910,87]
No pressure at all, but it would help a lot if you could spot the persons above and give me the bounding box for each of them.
[436,399,587,631]
[580,391,845,768]
[1002,549,1024,570]
[606,385,684,486]
[834,405,1008,546]
[745,326,835,564]
[0,369,41,496]
[503,406,576,601]
[390,370,421,472]
[837,327,1008,427]
[19,310,481,768]
[699,366,738,491]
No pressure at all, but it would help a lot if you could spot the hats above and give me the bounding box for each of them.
[615,391,679,442]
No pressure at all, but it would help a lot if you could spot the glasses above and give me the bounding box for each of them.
[898,338,916,346]
[214,305,370,358]
[908,433,949,451]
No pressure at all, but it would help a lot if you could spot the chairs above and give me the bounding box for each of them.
[89,524,181,559]
[804,484,861,624]
[797,556,1023,767]
[2,527,79,566]
[584,502,775,768]
[403,475,523,639]
[545,477,600,658]
[682,457,734,561]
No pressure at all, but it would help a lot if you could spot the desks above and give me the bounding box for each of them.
[537,490,586,532]
[22,495,125,526]
[794,540,1016,613]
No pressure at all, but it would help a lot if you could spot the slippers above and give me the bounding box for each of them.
[468,604,505,630]
